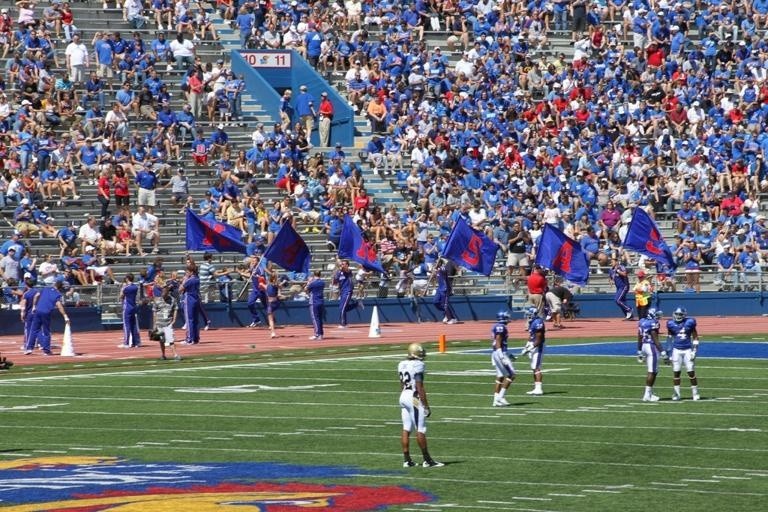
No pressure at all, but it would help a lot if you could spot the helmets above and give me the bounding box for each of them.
[647,306,663,319]
[408,344,425,360]
[527,307,539,319]
[496,311,512,322]
[673,306,687,321]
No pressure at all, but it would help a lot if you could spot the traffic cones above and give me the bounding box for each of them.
[59,320,76,357]
[366,303,385,338]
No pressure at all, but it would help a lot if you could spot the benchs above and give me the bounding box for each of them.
[1,0,768,305]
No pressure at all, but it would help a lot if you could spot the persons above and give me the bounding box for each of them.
[522,304,550,395]
[152,291,180,361]
[179,264,202,344]
[250,273,365,340]
[635,307,669,401]
[665,307,703,401]
[1,0,767,295]
[117,274,143,349]
[525,261,650,326]
[490,309,519,407]
[431,259,461,323]
[394,343,446,467]
[20,277,72,354]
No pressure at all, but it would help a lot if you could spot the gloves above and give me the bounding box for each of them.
[637,352,646,363]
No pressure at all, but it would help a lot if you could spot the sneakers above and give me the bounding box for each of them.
[643,394,659,401]
[423,461,445,467]
[627,308,633,318]
[24,350,32,355]
[447,318,457,324]
[672,393,681,401]
[118,344,129,348]
[250,321,261,327]
[497,396,510,405]
[693,392,700,401]
[526,390,543,395]
[403,460,419,467]
[309,334,322,340]
[443,316,448,322]
[205,320,211,330]
[359,300,365,310]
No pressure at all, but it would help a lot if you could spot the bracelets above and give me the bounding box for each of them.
[64,313,67,318]
